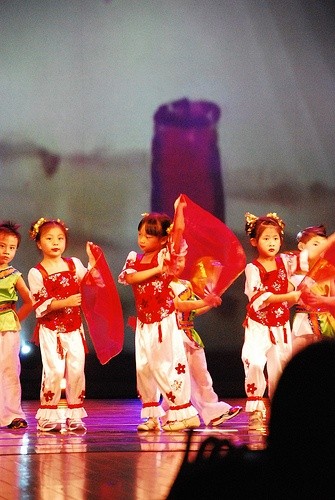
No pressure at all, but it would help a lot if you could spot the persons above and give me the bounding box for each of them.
[119,193,197,430]
[28,218,99,432]
[0,221,32,430]
[170,246,242,426]
[241,214,335,429]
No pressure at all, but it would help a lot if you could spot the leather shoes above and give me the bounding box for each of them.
[137,417,161,431]
[162,415,201,430]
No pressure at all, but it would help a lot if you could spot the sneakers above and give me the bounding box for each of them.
[248,411,264,424]
[212,405,243,426]
[9,418,27,429]
[65,418,88,431]
[37,418,62,431]
